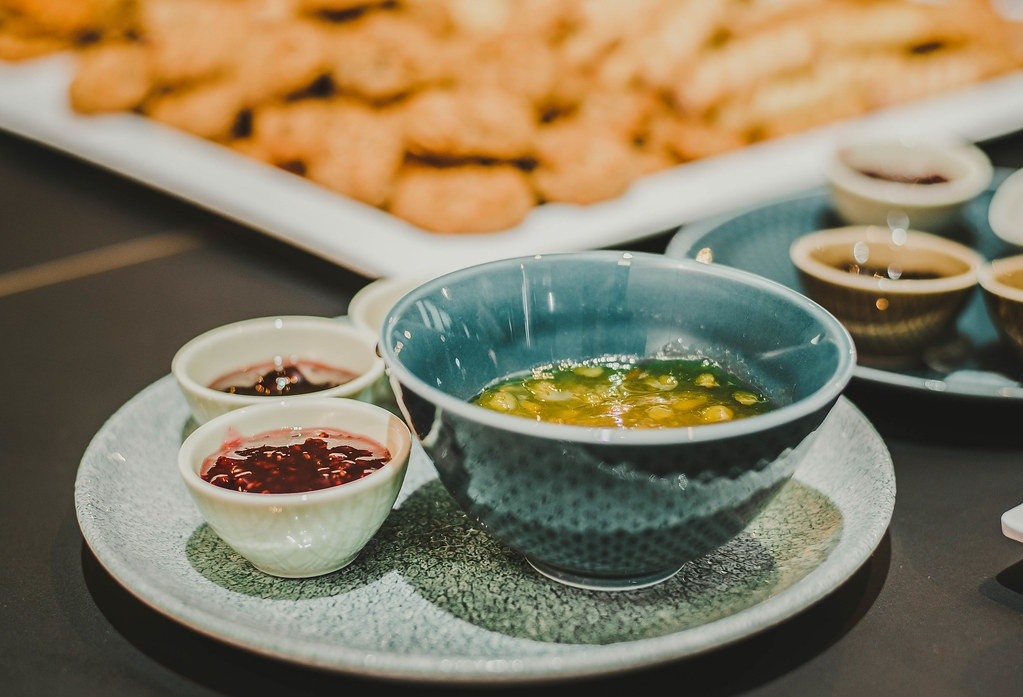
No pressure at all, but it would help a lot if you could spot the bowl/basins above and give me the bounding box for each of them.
[178,394,413,580]
[378,246,860,590]
[784,224,983,361]
[169,313,379,421]
[831,132,992,226]
[977,251,1023,358]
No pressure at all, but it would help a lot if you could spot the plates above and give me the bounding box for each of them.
[667,168,1023,402]
[75,366,898,688]
[4,51,1020,280]
